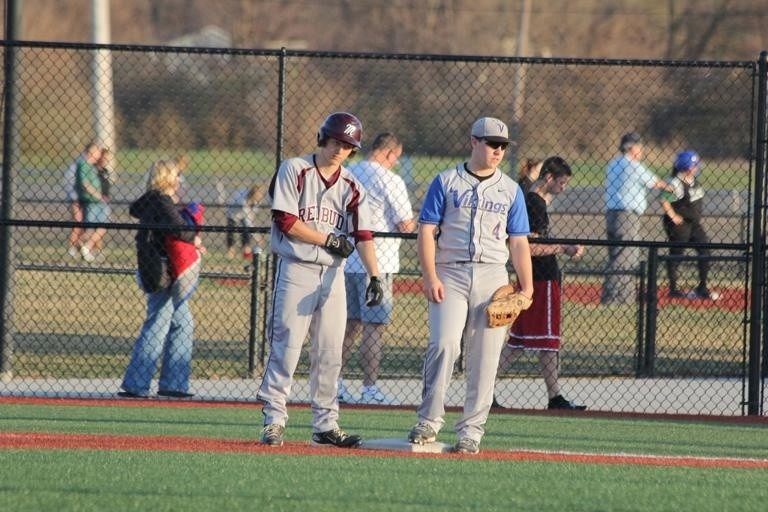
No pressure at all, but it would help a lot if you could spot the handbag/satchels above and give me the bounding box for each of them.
[136,223,174,293]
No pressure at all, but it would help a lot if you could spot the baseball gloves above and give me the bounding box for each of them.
[486,284,534,328]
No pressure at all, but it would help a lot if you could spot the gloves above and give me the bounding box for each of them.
[365,276,384,307]
[326,233,355,258]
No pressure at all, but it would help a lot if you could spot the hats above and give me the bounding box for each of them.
[469,117,517,145]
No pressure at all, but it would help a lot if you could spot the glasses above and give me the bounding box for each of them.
[485,141,507,150]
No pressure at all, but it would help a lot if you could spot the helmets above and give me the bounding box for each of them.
[317,112,363,159]
[674,152,701,167]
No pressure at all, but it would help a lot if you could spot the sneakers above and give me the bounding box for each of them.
[490,395,506,408]
[356,389,400,405]
[455,438,479,456]
[408,422,436,444]
[548,395,588,411]
[336,385,358,404]
[157,390,196,400]
[114,388,158,401]
[260,424,286,447]
[311,429,362,450]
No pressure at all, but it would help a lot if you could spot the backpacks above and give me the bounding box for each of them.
[136,213,171,293]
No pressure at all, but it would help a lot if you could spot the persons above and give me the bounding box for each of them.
[224,186,266,262]
[144,156,188,206]
[659,151,723,303]
[601,132,672,306]
[119,160,200,399]
[261,112,385,448]
[93,148,111,205]
[409,116,533,455]
[514,155,553,208]
[64,153,93,258]
[78,142,110,262]
[482,156,586,410]
[345,133,418,408]
